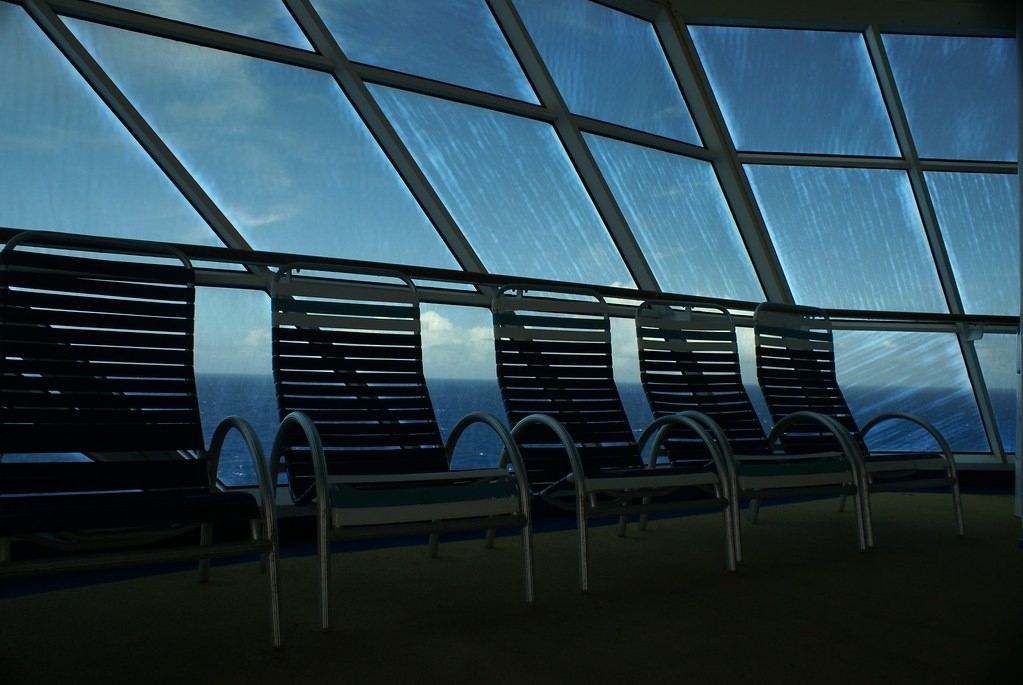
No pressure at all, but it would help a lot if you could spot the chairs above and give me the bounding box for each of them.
[635,299,866,563]
[259,261,534,635]
[749,301,966,548]
[486,283,737,594]
[1,230,281,649]
